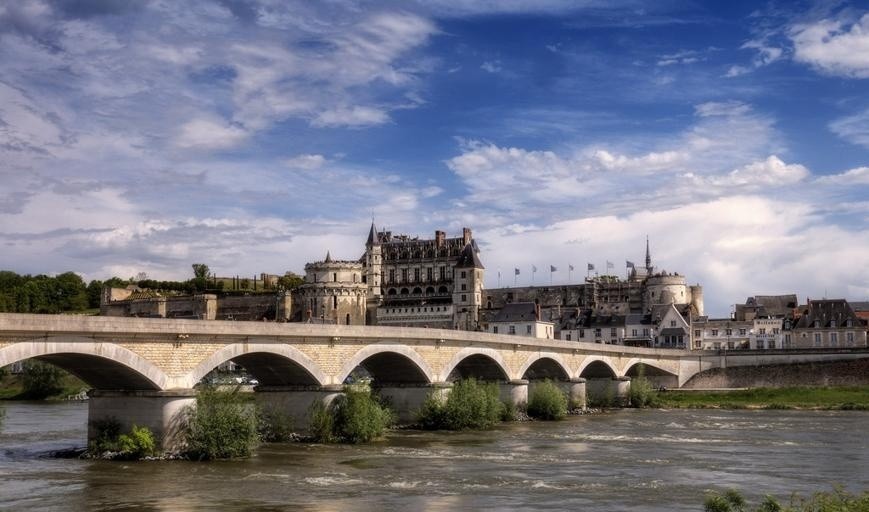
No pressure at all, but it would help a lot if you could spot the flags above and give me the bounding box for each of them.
[515,268,520,275]
[532,266,536,272]
[551,265,557,272]
[607,262,614,268]
[626,261,634,267]
[569,265,573,271]
[588,263,595,270]
